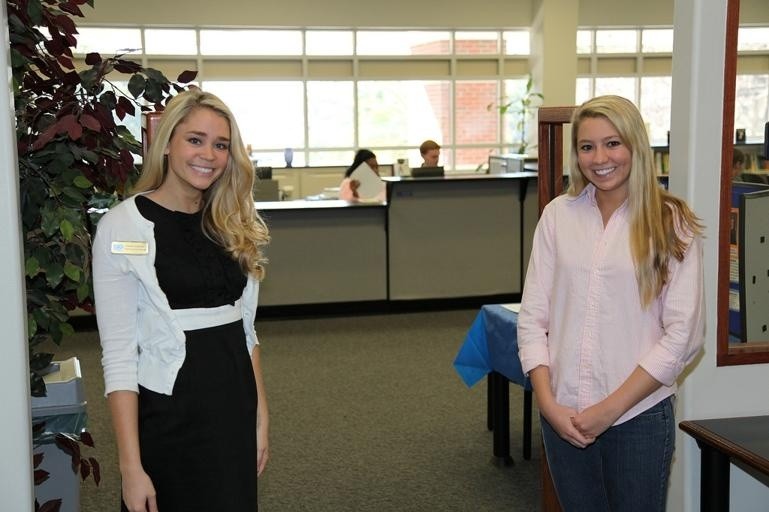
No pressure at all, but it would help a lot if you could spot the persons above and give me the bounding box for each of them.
[88,91,274,512]
[728,215,736,244]
[759,156,769,170]
[731,146,747,184]
[339,150,386,203]
[516,93,710,511]
[419,139,442,169]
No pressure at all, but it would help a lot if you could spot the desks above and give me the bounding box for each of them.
[676,410,768,512]
[242,152,570,324]
[477,298,554,463]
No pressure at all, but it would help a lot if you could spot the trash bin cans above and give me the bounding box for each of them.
[29,357,87,512]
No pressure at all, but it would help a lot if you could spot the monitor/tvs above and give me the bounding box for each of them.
[256,167,272,178]
[410,166,445,176]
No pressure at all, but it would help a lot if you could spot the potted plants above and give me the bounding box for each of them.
[1,0,208,512]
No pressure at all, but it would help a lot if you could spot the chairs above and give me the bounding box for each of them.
[253,177,285,203]
